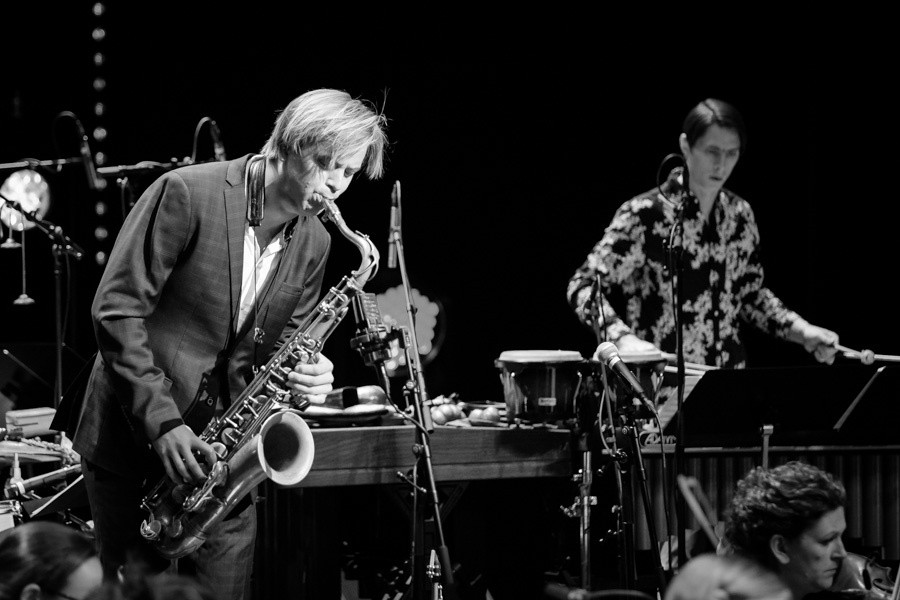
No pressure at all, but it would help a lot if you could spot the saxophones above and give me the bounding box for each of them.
[139,196,381,561]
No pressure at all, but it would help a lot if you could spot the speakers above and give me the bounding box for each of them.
[256,420,577,571]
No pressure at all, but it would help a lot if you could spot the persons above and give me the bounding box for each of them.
[564,99,839,443]
[0,523,216,599]
[76,87,386,600]
[660,462,849,599]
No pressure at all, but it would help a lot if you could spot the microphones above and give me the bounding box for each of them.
[596,340,659,416]
[386,181,400,269]
[26,209,87,264]
